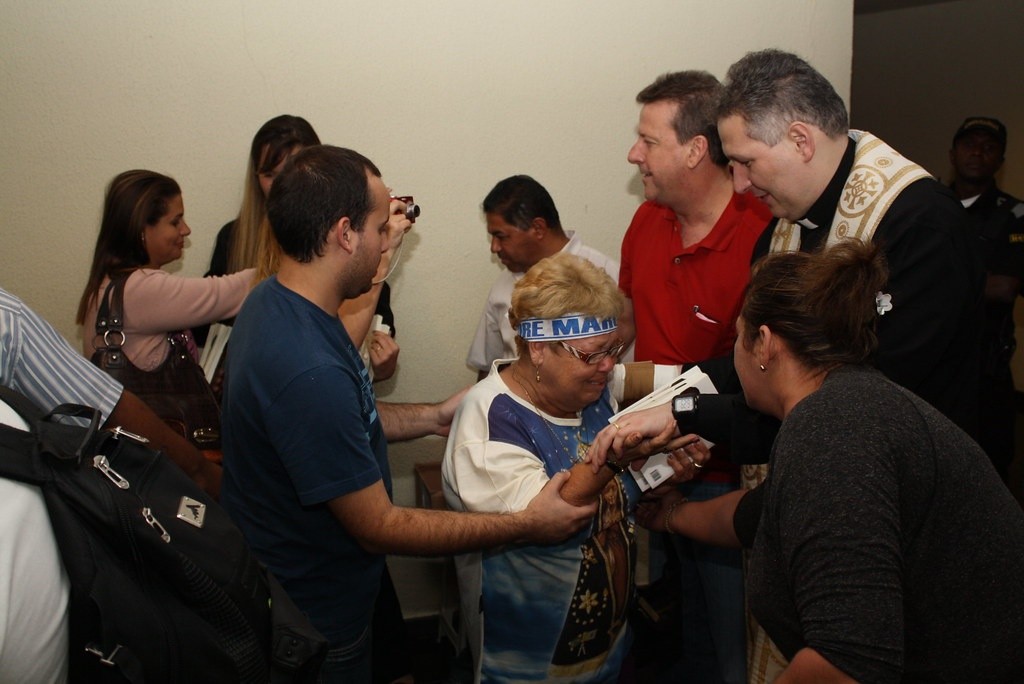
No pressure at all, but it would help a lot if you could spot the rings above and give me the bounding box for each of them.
[694,463,703,468]
[376,346,381,353]
[613,423,620,430]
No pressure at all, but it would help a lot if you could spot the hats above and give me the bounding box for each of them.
[953,117,1007,158]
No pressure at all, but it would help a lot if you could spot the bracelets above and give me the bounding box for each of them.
[663,497,690,535]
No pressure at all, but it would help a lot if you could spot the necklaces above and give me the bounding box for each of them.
[510,360,579,465]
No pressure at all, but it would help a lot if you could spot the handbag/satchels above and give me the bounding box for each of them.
[90,269,223,451]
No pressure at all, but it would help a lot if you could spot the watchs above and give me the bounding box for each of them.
[671,386,702,435]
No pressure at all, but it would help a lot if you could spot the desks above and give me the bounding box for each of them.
[415,463,468,656]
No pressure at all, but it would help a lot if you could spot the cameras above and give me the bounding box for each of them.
[390,195,420,223]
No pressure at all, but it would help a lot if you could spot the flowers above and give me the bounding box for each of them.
[876,291,893,316]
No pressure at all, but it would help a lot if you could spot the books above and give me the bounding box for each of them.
[607,365,719,493]
[198,322,234,386]
[359,315,392,384]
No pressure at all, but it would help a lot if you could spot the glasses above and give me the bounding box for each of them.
[556,340,625,365]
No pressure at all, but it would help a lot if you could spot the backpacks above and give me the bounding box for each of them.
[0,384,330,684]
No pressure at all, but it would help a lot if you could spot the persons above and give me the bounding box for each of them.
[630,234,1024,684]
[944,115,1024,465]
[74,169,279,452]
[615,68,772,684]
[467,174,634,384]
[0,399,72,684]
[217,144,599,683]
[188,113,411,381]
[0,286,223,500]
[435,250,711,684]
[583,47,1024,684]
